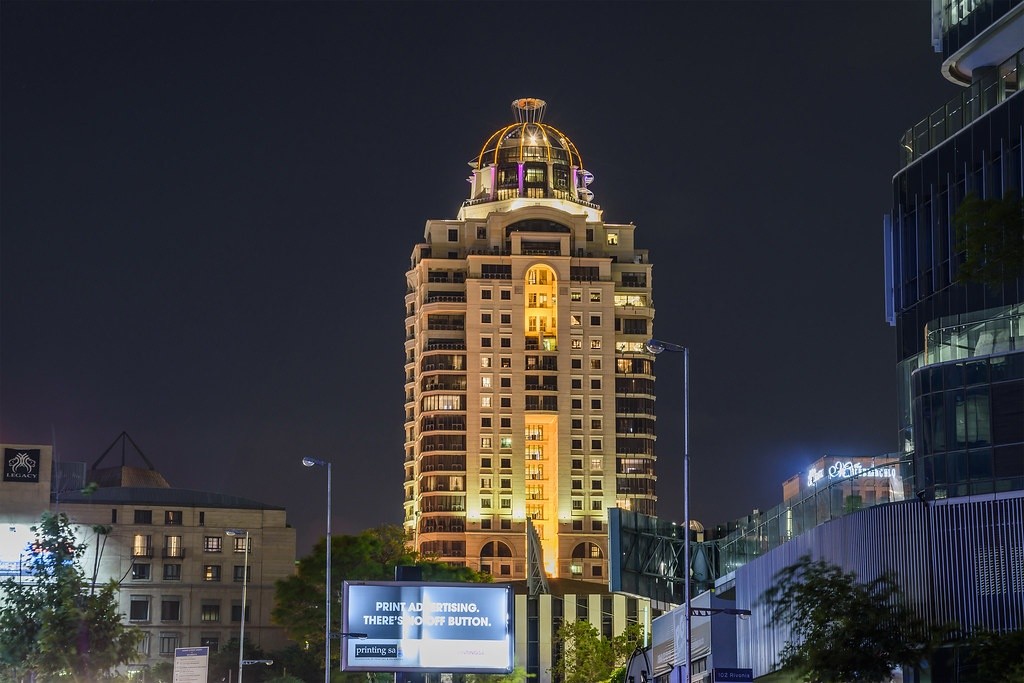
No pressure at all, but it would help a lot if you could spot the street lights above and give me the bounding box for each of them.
[225,529,273,683]
[917,490,937,626]
[20,554,30,592]
[644,341,752,683]
[301,457,368,683]
